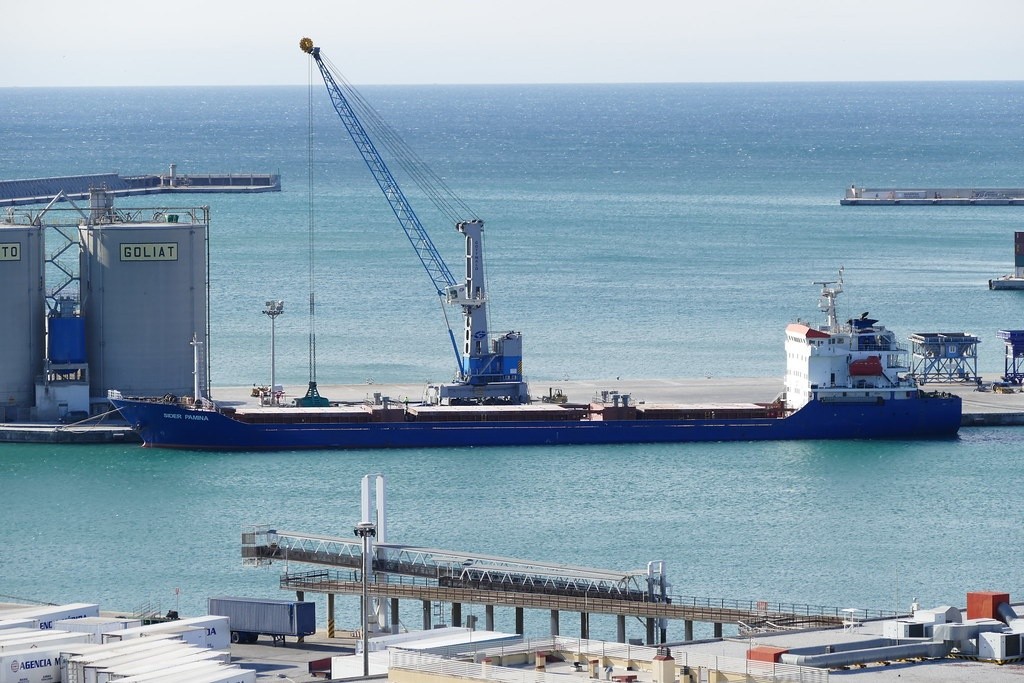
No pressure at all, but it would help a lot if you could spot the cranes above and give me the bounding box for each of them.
[299,38,532,406]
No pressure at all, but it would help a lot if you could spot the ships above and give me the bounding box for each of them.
[106,264,963,450]
